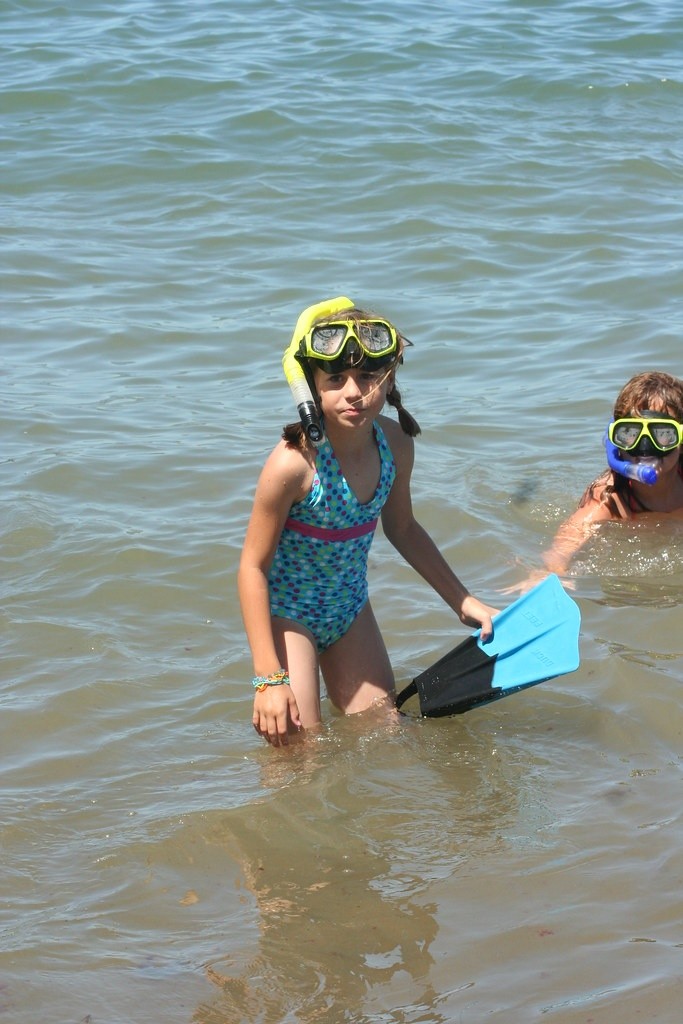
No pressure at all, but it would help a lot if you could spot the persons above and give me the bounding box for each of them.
[237,296,502,747]
[571,371,683,521]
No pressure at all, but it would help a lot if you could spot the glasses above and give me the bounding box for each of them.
[298,319,397,361]
[609,416,683,452]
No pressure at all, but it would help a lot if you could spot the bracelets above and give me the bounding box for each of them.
[252,669,291,692]
